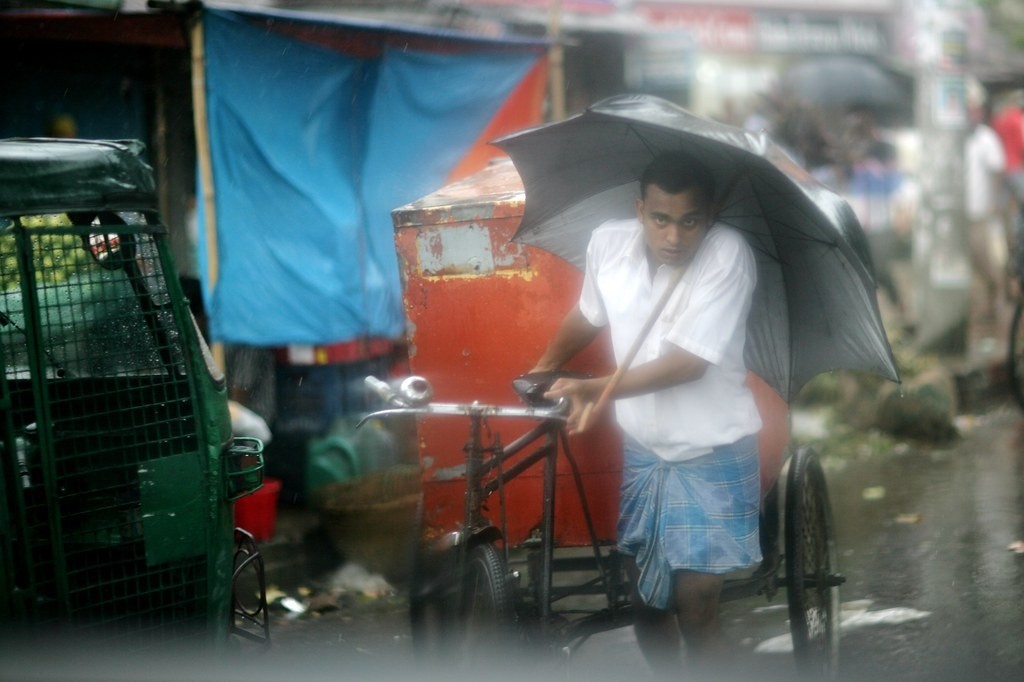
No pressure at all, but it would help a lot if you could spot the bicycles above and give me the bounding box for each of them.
[1003,220,1024,406]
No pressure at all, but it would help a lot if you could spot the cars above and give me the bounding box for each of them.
[641,50,910,120]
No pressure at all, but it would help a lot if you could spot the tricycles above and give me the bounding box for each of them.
[353,160,844,682]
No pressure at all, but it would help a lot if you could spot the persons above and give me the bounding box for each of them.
[963,75,1024,360]
[529,153,765,682]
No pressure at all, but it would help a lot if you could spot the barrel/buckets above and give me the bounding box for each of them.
[234,481,279,542]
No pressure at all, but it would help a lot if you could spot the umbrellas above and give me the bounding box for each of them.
[486,92,902,404]
